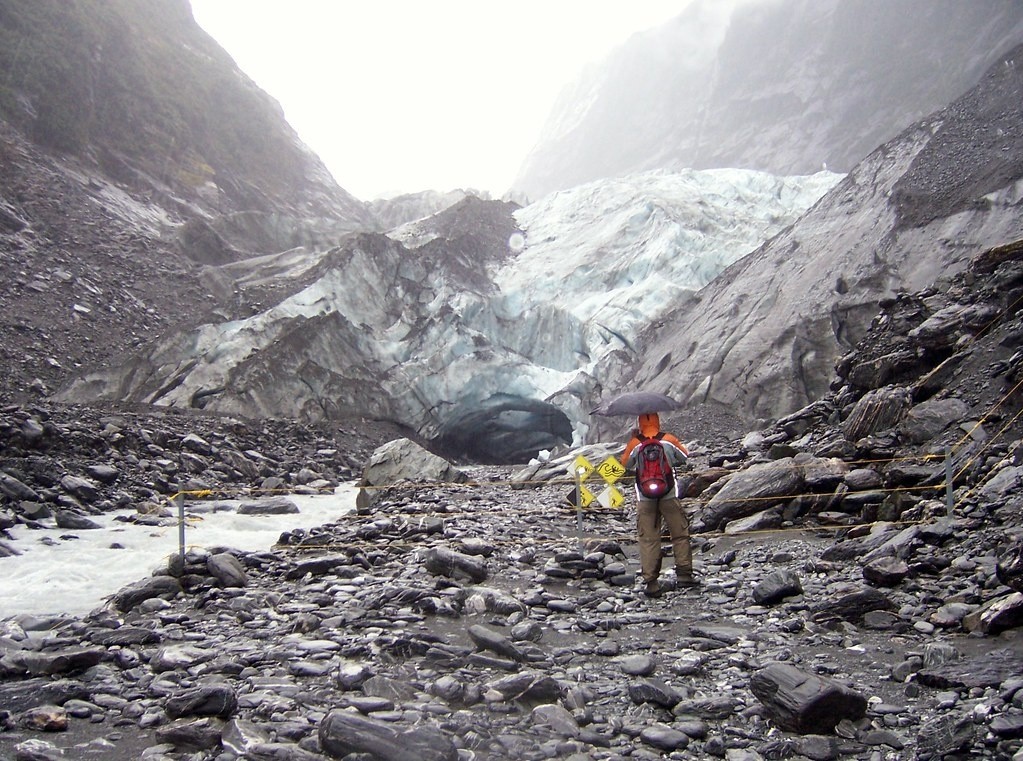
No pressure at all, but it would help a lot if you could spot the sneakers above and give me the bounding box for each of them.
[677,576,700,587]
[644,582,661,598]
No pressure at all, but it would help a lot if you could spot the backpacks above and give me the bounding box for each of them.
[635,432,674,498]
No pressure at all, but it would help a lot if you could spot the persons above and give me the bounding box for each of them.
[621,413,701,598]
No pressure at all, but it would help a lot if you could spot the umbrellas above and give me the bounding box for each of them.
[589,389,684,435]
